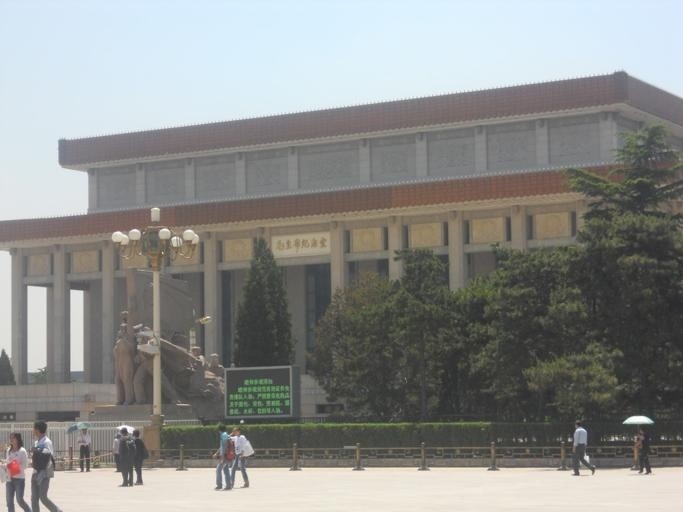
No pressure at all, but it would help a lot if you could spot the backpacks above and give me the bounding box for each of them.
[226,437,236,462]
[127,440,137,459]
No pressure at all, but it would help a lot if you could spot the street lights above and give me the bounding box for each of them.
[112,207,199,449]
[189,316,212,352]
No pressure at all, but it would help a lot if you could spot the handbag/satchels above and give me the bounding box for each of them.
[242,440,256,458]
[0,460,21,483]
[580,453,590,466]
[142,448,150,459]
[31,446,53,474]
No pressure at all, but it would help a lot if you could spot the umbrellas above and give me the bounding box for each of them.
[622,415,654,433]
[117,425,135,434]
[66,422,93,432]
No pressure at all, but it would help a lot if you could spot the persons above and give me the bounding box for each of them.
[112,428,145,487]
[211,425,231,491]
[572,421,595,476]
[0,432,31,512]
[228,427,248,488]
[78,429,92,472]
[30,421,64,512]
[632,428,652,475]
[112,310,226,406]
